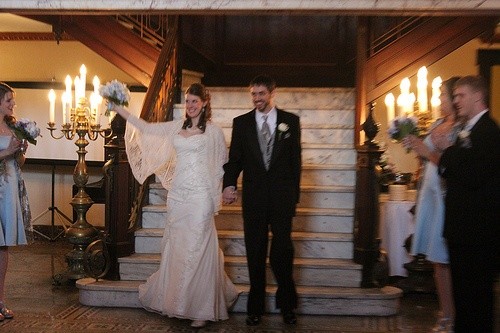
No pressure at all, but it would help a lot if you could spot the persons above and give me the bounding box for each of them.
[221,74,303,326]
[0,81,29,320]
[430,75,500,333]
[103,84,239,327]
[400,75,462,333]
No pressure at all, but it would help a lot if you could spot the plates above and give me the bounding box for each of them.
[379,184,417,203]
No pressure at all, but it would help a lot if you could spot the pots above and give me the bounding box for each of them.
[382,172,413,185]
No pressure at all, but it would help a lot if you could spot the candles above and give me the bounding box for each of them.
[47,63,121,127]
[384,65,443,127]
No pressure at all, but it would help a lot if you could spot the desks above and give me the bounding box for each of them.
[379,199,430,286]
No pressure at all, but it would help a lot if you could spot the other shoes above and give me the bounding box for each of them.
[0,308,14,321]
[190,321,206,327]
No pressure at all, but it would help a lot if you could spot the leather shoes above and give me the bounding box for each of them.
[280,309,296,324]
[247,315,261,325]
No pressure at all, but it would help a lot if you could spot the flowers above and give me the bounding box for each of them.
[278,123,289,136]
[12,118,43,151]
[458,130,471,139]
[100,80,131,109]
[387,117,421,153]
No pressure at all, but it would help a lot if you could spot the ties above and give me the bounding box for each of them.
[261,116,271,141]
